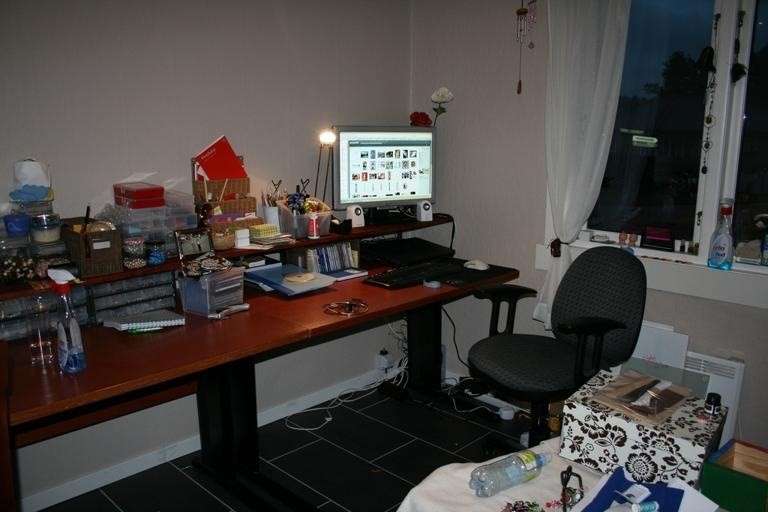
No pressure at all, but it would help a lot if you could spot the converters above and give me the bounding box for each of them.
[499,407,515,420]
[377,381,409,402]
[383,357,394,369]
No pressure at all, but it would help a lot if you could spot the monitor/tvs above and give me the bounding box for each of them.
[331,125,438,211]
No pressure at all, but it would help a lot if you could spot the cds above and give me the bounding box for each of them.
[285,272,314,281]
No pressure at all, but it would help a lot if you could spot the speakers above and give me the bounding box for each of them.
[346,205,365,228]
[416,199,433,222]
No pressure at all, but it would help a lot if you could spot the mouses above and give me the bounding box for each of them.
[463,259,491,271]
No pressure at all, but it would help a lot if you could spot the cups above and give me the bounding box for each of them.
[28,313,57,365]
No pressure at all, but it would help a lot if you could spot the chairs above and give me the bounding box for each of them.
[467,247,647,452]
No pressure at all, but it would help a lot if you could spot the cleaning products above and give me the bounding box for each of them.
[707,198,738,271]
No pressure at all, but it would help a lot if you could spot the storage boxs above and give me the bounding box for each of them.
[552,368,728,499]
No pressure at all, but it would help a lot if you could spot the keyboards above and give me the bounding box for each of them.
[364,259,463,291]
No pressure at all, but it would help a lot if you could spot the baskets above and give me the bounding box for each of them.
[194,178,263,231]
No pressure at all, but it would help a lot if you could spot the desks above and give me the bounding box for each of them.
[2,213,521,511]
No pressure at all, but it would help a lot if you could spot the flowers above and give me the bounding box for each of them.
[409,85,455,136]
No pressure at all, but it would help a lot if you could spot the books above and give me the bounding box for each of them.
[103,309,186,334]
[196,134,247,182]
[233,241,369,296]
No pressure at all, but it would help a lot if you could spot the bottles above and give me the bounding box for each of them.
[704,392,721,416]
[308,207,320,240]
[469,450,552,497]
[31,214,61,243]
[123,237,166,269]
